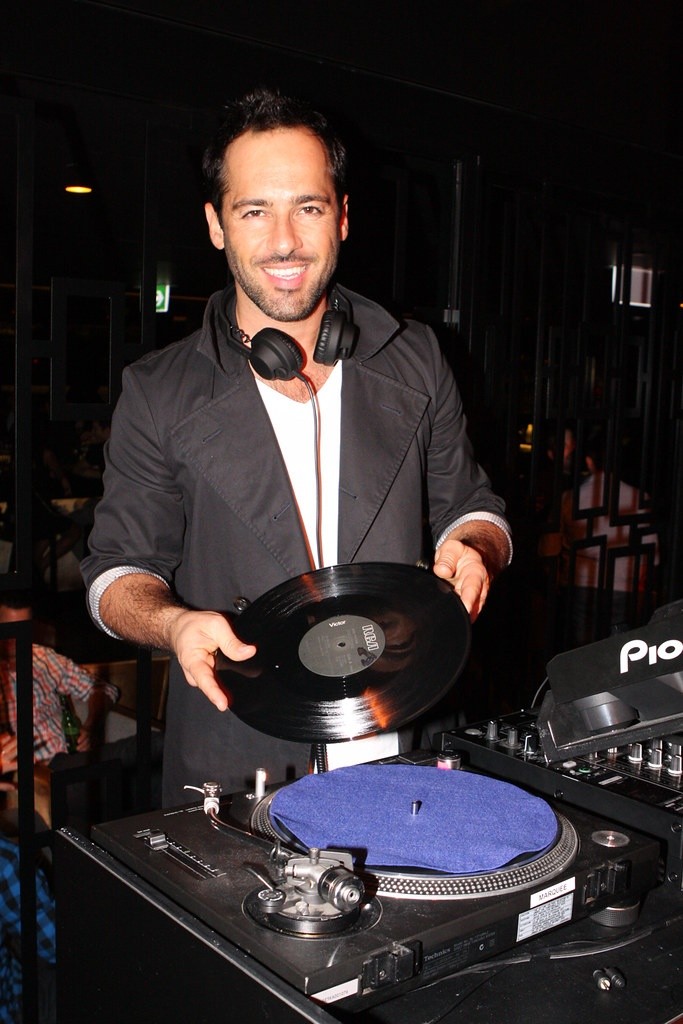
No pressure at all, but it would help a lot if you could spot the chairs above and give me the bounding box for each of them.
[5,693,160,829]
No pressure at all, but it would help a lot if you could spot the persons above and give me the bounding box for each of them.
[0,838,57,1024]
[80,89,516,810]
[0,570,119,774]
[0,385,683,596]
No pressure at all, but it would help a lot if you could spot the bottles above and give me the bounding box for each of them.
[59,695,80,753]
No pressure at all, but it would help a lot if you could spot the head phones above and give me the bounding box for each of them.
[217,278,359,382]
